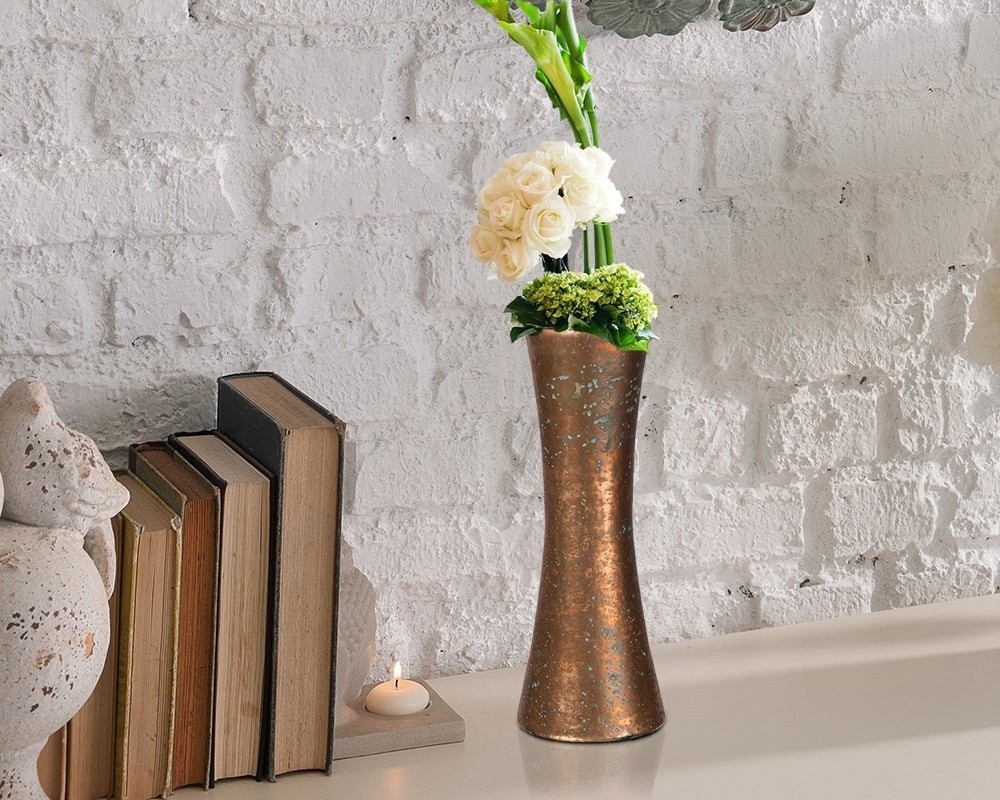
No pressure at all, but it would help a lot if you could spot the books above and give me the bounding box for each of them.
[125,440,222,800]
[67,510,118,800]
[37,724,68,800]
[168,427,275,788]
[214,372,347,782]
[103,467,183,800]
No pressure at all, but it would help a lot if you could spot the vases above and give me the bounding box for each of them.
[506,324,673,745]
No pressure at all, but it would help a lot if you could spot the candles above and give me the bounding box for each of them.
[368,660,435,732]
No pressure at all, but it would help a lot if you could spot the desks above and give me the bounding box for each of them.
[142,588,1000,800]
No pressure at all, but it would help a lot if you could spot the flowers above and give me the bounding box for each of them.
[456,1,661,355]
